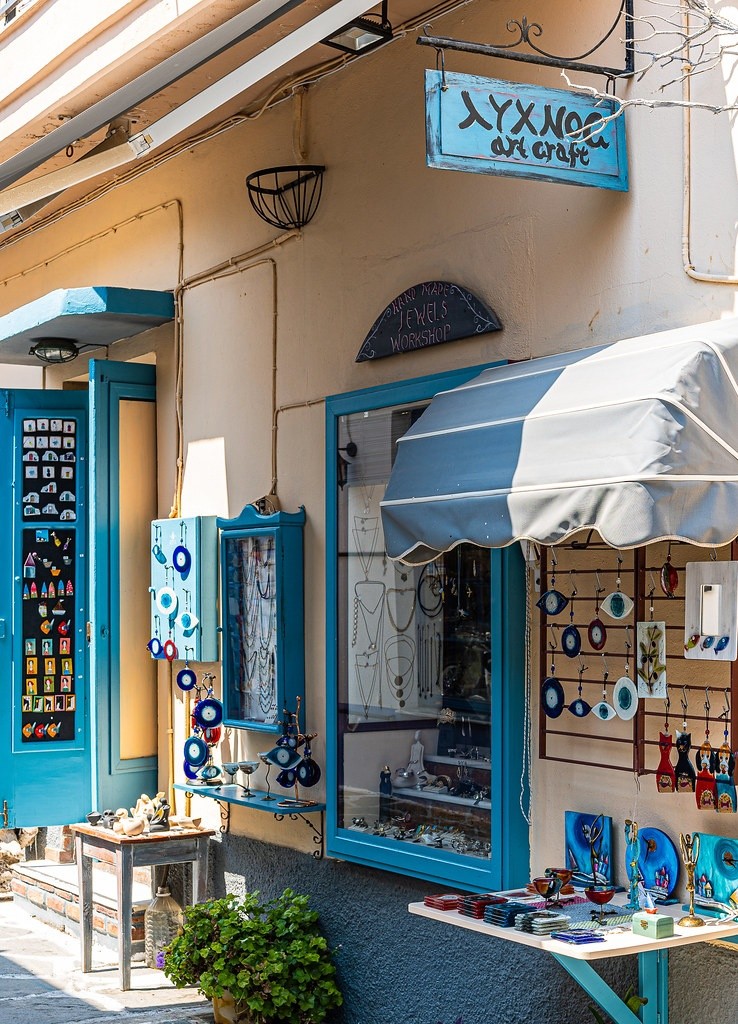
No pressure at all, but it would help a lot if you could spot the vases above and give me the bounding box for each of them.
[212,988,256,1024]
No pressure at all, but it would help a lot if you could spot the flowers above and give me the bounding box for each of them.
[155,887,343,1024]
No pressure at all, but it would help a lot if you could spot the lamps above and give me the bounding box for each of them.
[34,340,78,363]
[320,18,393,56]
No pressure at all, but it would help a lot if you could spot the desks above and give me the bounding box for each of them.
[408,888,738,1024]
[68,823,217,992]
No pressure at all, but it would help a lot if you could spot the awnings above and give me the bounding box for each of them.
[379,316,738,567]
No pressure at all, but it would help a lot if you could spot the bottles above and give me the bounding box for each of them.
[144,885,184,970]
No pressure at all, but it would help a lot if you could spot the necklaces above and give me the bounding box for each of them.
[237,538,275,714]
[351,484,445,720]
